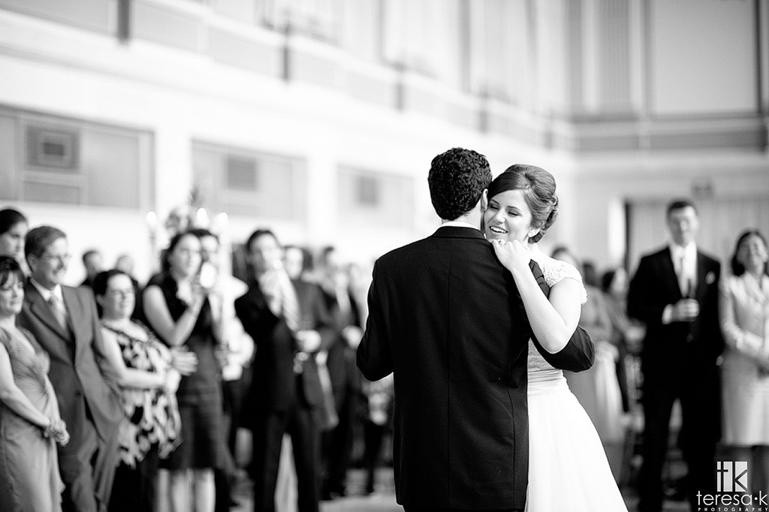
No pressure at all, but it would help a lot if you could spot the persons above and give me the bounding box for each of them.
[476,165,641,511]
[357,148,596,511]
[1,200,768,511]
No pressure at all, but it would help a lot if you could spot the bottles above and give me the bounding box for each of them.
[684,278,698,323]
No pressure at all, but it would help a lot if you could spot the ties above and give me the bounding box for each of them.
[677,257,689,300]
[278,288,300,334]
[48,293,68,328]
